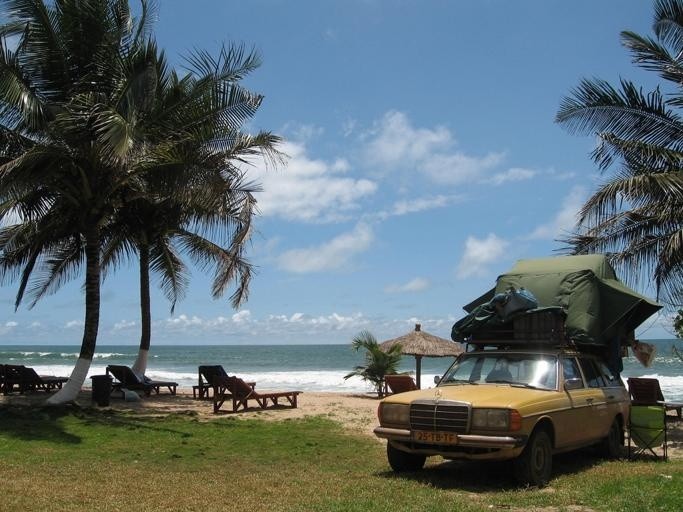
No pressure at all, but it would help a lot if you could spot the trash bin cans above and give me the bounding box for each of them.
[89,375,114,407]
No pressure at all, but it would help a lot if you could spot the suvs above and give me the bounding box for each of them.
[373,309,632,488]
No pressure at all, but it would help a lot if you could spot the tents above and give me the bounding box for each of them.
[462,254,664,348]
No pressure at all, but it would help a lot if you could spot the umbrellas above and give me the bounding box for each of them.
[376,323,466,390]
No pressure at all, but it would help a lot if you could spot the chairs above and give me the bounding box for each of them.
[199,365,256,401]
[486,369,512,385]
[626,403,667,462]
[384,375,419,396]
[626,377,683,416]
[0,364,69,395]
[213,374,303,413]
[106,365,178,398]
